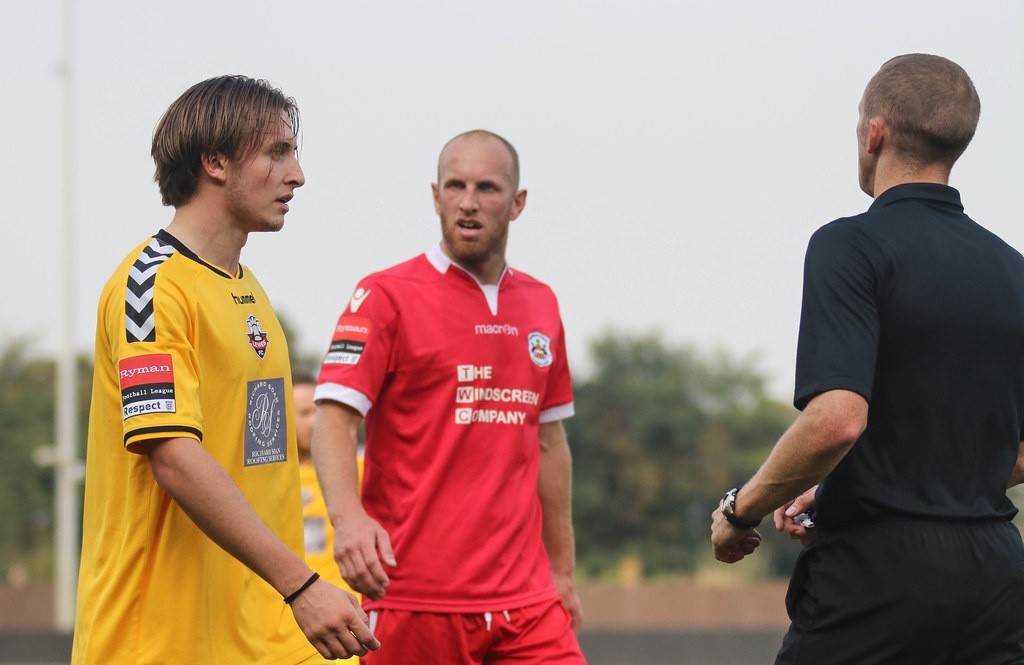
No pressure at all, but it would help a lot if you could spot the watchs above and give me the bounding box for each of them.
[721,488,763,531]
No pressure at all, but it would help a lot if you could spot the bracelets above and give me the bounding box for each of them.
[283,573,321,604]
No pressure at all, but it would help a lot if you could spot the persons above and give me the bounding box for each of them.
[293,377,366,665]
[711,53,1024,665]
[308,130,591,665]
[70,75,381,664]
